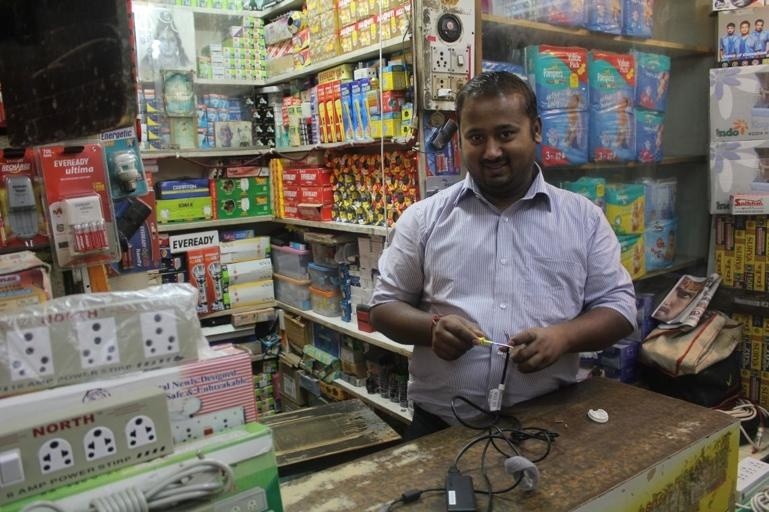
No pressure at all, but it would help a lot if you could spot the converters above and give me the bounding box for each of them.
[444,476,476,512]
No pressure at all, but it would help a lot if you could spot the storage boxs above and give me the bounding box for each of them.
[283,165,334,221]
[709,141,769,214]
[597,342,639,385]
[707,68,769,140]
[620,285,666,345]
[636,346,741,409]
[202,324,257,353]
[153,173,275,316]
[271,230,357,410]
[263,0,411,77]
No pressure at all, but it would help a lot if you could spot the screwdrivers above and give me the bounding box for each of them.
[479,337,513,350]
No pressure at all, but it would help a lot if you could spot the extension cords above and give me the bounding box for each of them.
[736,457,769,504]
[196,485,269,511]
[170,406,246,445]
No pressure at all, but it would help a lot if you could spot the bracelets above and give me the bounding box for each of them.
[429,312,440,337]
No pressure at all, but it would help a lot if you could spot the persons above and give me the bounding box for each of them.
[368,69,638,439]
[653,276,702,321]
[140,11,193,81]
[720,18,769,67]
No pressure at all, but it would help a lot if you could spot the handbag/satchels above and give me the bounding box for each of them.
[636,309,745,407]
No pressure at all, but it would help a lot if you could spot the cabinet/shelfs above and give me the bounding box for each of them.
[474,0,709,374]
[134,1,279,420]
[706,2,768,410]
[261,0,423,427]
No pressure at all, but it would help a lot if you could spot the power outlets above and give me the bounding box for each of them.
[430,45,450,74]
[432,75,452,102]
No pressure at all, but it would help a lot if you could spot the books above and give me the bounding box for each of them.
[651,272,723,333]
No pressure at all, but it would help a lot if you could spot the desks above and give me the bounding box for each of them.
[279,373,741,512]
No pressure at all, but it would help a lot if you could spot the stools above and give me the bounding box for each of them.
[258,395,407,474]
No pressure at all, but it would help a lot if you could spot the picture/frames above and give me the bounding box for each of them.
[159,67,196,118]
[170,119,198,151]
